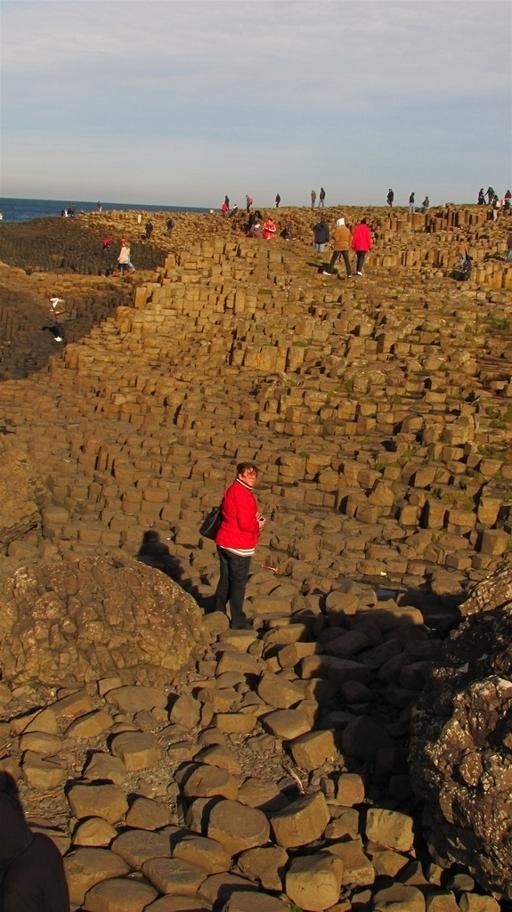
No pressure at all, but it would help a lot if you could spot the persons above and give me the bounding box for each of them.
[62,208,75,218]
[311,187,326,210]
[477,185,512,221]
[280,227,290,241]
[96,200,103,212]
[313,216,374,277]
[506,227,512,257]
[409,192,429,215]
[425,214,433,235]
[145,219,153,239]
[166,217,173,238]
[213,461,266,629]
[103,233,135,278]
[275,192,280,207]
[221,194,276,239]
[456,238,468,267]
[386,188,394,207]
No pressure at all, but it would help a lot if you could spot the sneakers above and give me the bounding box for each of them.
[231,622,253,630]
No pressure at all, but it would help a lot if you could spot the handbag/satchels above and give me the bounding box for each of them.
[200,496,224,539]
